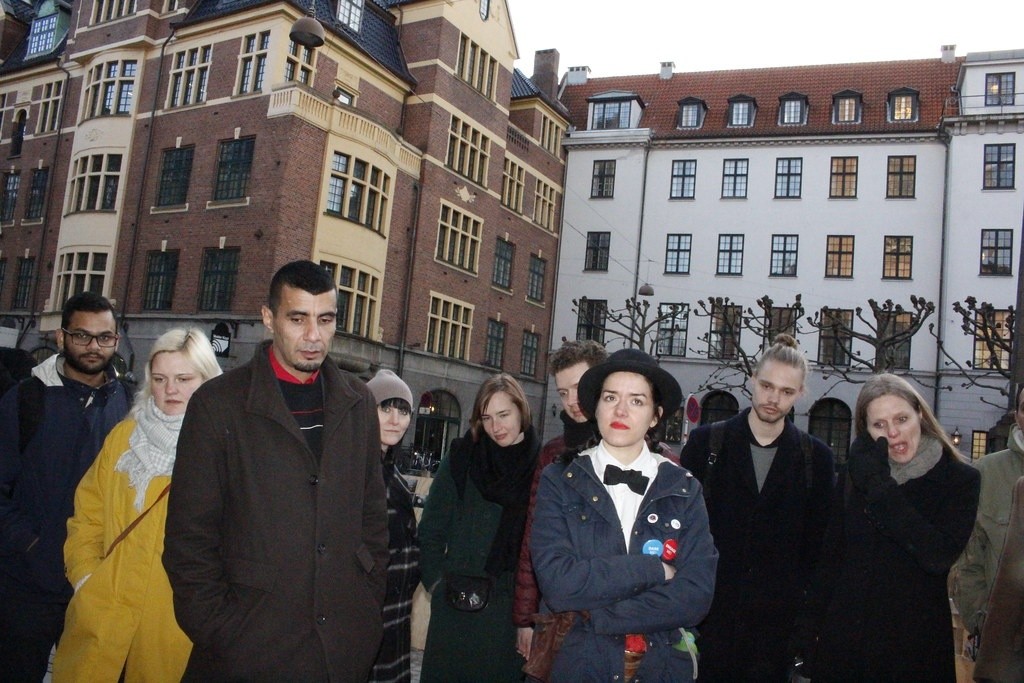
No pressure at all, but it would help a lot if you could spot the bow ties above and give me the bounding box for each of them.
[604,465,650,495]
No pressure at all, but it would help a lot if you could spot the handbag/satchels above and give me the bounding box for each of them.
[445,574,492,612]
[521,610,591,683]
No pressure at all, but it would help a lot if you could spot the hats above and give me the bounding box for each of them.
[577,348,682,426]
[365,368,414,415]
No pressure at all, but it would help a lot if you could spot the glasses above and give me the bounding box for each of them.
[61,327,118,347]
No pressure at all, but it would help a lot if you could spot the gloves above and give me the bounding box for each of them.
[848,431,897,505]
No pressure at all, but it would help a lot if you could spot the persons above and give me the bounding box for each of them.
[50,328,228,682]
[835,372,981,683]
[0,291,140,683]
[679,334,843,681]
[417,371,542,682]
[160,260,390,683]
[515,339,717,682]
[947,383,1024,683]
[364,368,422,683]
